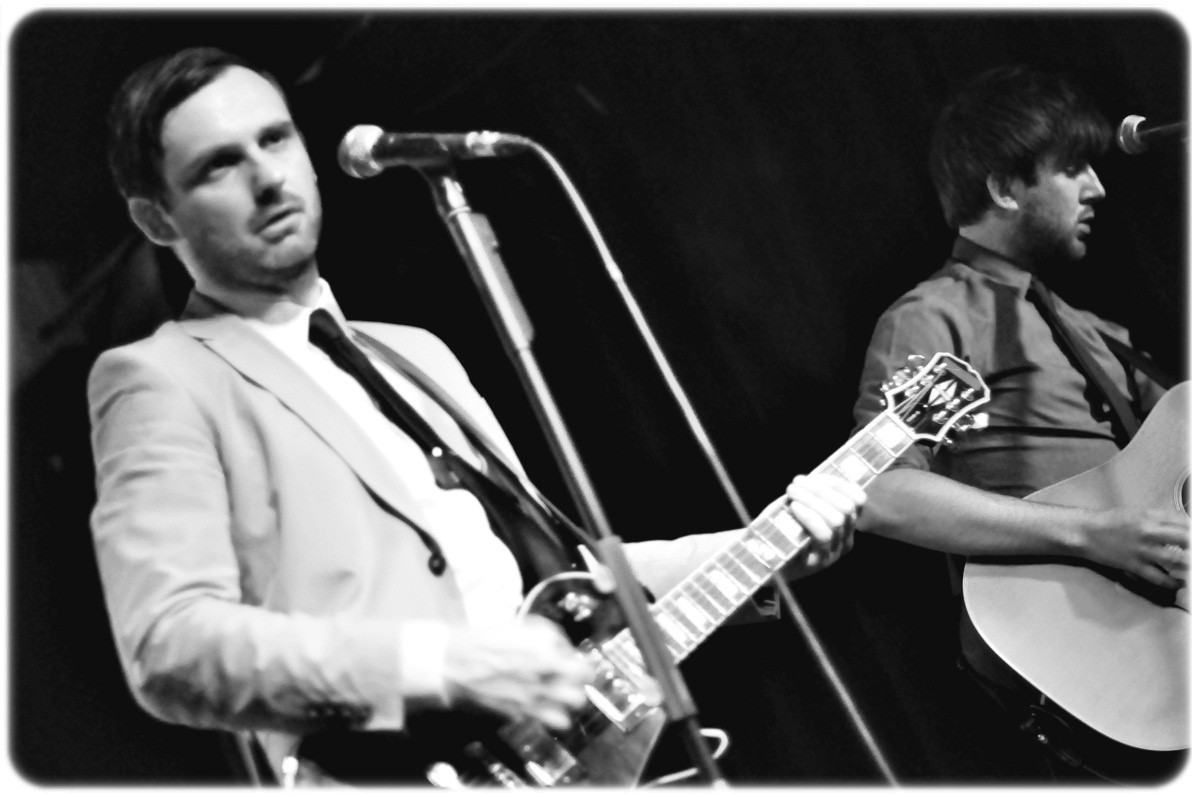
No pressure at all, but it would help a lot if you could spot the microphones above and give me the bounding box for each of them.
[338,123,533,178]
[1116,112,1189,155]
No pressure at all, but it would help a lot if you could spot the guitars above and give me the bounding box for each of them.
[240,352,992,788]
[958,380,1191,787]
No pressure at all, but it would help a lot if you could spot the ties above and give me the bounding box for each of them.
[1025,277,1139,445]
[309,306,596,646]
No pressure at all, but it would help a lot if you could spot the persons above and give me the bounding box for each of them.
[851,65,1191,782]
[89,49,866,788]
